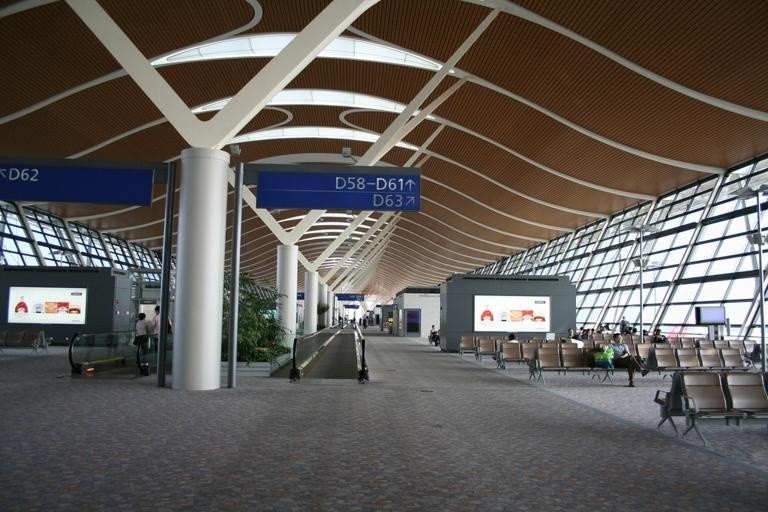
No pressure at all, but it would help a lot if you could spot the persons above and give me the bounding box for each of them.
[150,306,172,353]
[133,312,152,354]
[429,325,441,346]
[599,333,650,387]
[493,332,520,370]
[567,315,669,343]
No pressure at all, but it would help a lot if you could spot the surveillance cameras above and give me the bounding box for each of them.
[230,145,240,156]
[341,147,352,158]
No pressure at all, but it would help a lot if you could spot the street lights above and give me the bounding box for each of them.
[621,219,659,344]
[48,250,73,266]
[523,260,545,274]
[729,182,768,374]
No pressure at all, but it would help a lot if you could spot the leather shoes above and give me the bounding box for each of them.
[641,369,648,376]
[628,384,636,387]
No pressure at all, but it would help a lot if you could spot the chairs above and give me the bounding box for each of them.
[0,329,48,357]
[460,332,768,446]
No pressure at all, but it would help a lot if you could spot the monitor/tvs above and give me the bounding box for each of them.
[695,306,725,325]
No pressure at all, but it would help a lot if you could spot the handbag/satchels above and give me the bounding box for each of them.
[591,348,614,369]
